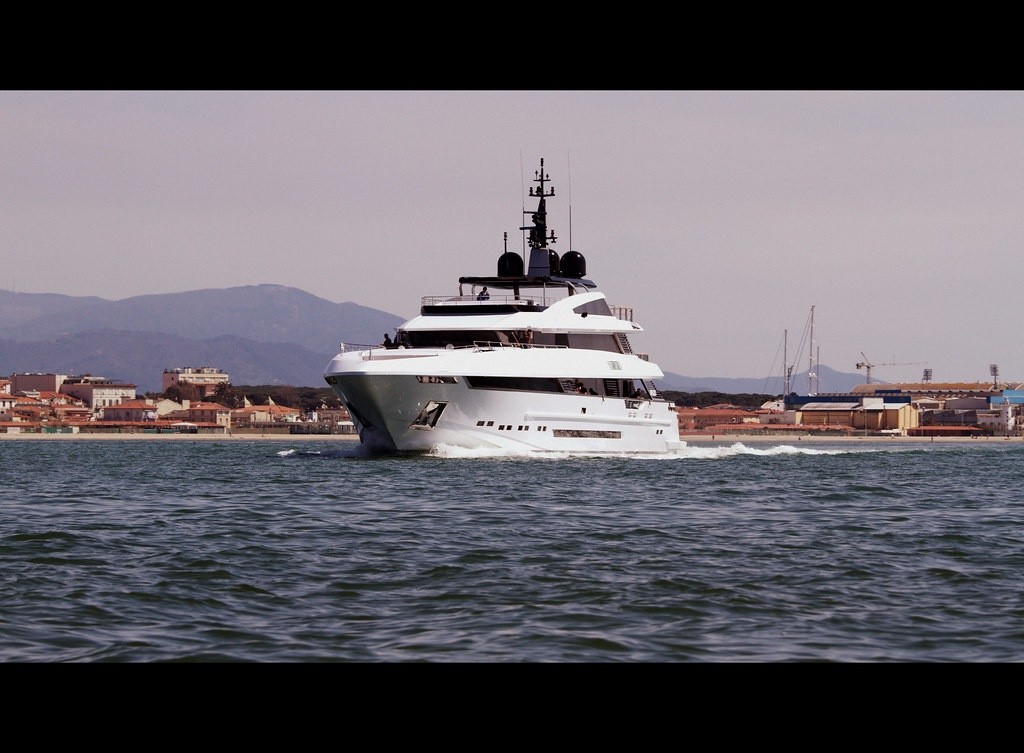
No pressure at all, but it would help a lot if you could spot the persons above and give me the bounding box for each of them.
[477,287,490,301]
[635,388,641,398]
[382,334,397,347]
[577,383,599,396]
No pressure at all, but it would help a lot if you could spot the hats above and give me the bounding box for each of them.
[637,388,641,390]
[526,329,532,332]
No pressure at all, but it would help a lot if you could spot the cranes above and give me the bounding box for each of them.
[855,350,930,385]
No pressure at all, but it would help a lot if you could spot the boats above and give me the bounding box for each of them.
[318,149,689,461]
